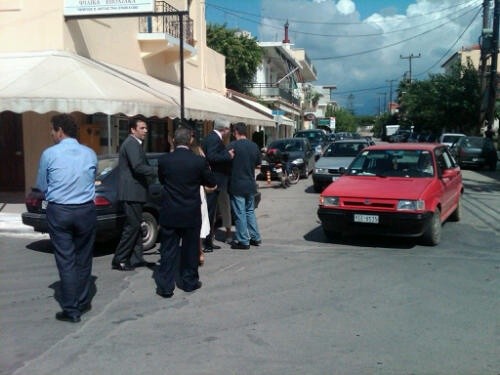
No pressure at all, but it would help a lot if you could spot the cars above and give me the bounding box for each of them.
[316,141,465,247]
[292,128,376,159]
[437,133,471,150]
[389,130,435,143]
[260,138,315,179]
[21,151,262,251]
[313,140,376,189]
[450,137,498,171]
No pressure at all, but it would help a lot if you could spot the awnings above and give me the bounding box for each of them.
[0,50,298,126]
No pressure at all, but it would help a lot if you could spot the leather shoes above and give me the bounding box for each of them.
[56,312,80,322]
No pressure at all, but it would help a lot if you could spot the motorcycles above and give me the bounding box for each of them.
[275,153,300,188]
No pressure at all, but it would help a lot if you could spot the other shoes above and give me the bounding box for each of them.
[208,244,220,249]
[185,282,201,292]
[250,239,261,246]
[231,242,250,249]
[133,261,156,267]
[111,262,134,271]
[156,288,173,297]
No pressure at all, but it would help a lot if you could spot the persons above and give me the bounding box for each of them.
[485,125,494,138]
[417,128,436,143]
[37,115,99,322]
[226,122,263,250]
[155,117,235,298]
[110,116,158,271]
